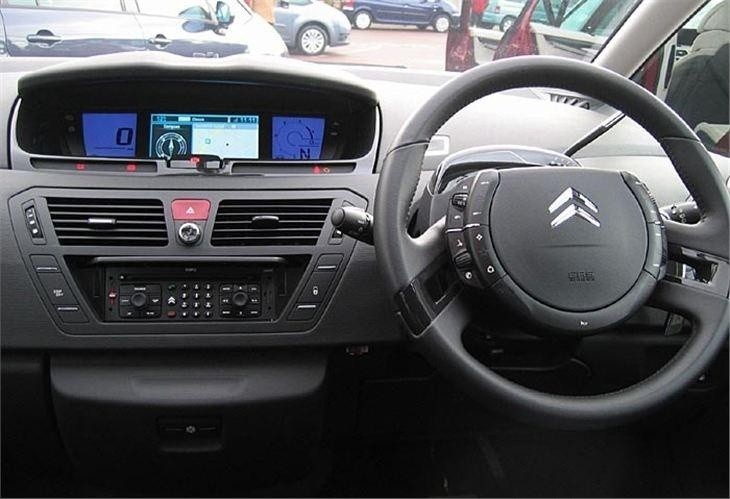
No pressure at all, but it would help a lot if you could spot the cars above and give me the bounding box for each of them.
[476,0,722,102]
